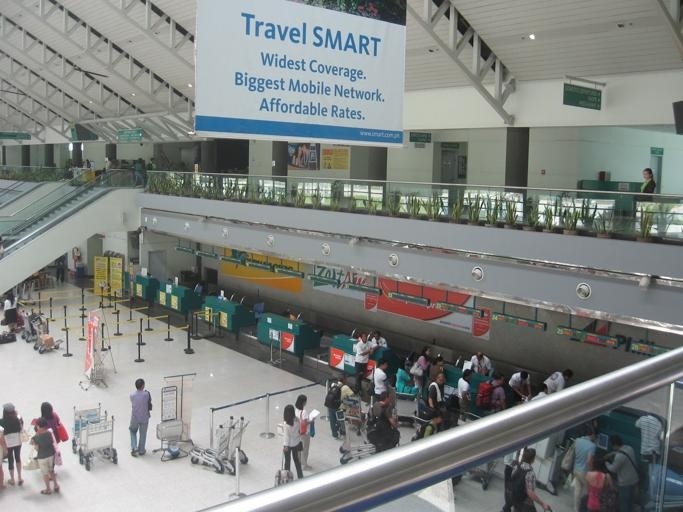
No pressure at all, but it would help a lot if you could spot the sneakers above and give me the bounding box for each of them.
[8,479,24,486]
[41,486,61,495]
[131,450,146,457]
[301,465,312,471]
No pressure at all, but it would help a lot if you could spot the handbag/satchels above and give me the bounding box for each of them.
[409,362,423,376]
[21,424,69,470]
[561,446,647,511]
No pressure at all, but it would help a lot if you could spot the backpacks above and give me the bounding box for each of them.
[476,383,493,407]
[361,378,375,395]
[325,386,341,409]
[367,417,399,448]
[505,462,529,504]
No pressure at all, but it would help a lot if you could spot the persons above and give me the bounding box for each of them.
[0,402,25,487]
[0,235,6,261]
[105,157,157,187]
[282,395,316,480]
[634,167,657,202]
[0,423,10,491]
[324,330,572,451]
[29,400,60,481]
[289,144,303,168]
[634,405,663,493]
[570,429,641,511]
[28,416,61,495]
[128,378,153,459]
[2,291,19,334]
[513,448,551,512]
[85,159,91,170]
[53,255,67,285]
[299,143,310,166]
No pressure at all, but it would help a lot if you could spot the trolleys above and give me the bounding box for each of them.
[14,281,35,306]
[186,416,250,476]
[17,310,64,354]
[463,460,499,491]
[70,403,118,472]
[394,387,420,428]
[333,403,376,466]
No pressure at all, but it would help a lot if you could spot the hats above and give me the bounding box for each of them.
[2,402,15,412]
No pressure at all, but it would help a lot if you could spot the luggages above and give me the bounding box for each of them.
[275,470,293,485]
